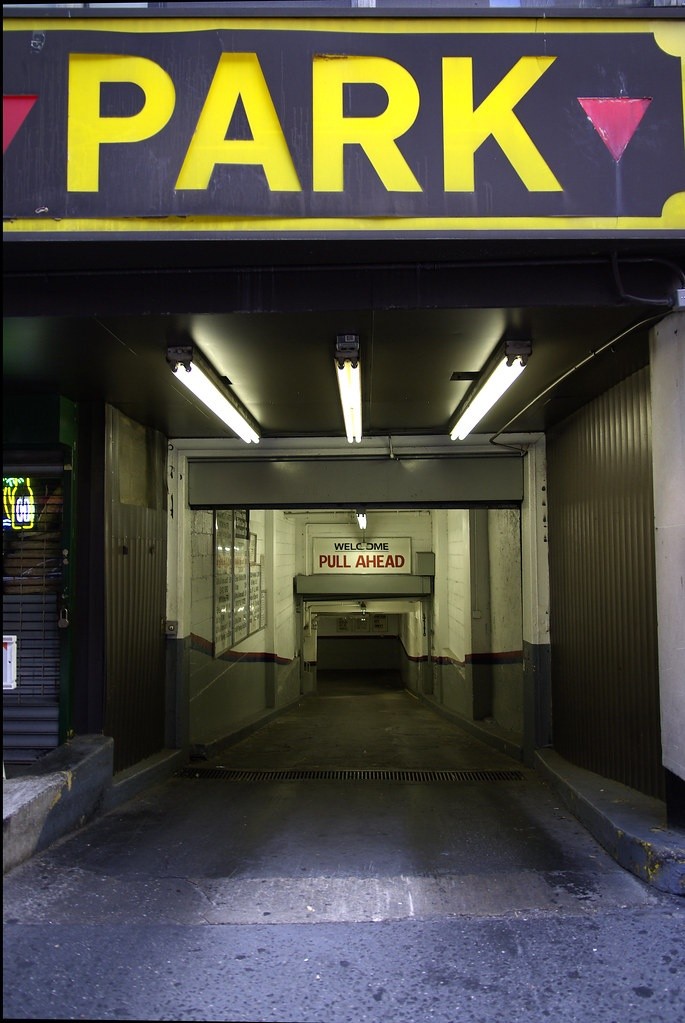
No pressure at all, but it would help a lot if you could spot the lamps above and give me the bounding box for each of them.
[447,340,533,441]
[356,508,367,530]
[164,344,264,445]
[333,333,364,444]
